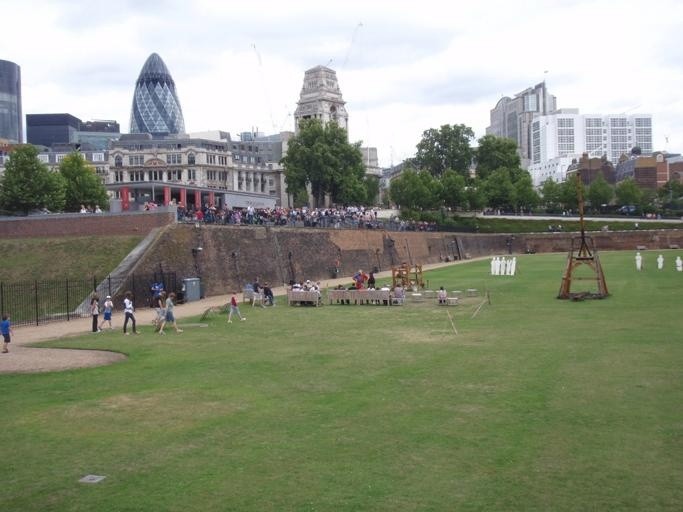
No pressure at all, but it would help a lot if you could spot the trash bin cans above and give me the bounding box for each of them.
[176,292,185,304]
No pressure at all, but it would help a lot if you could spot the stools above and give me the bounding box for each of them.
[391,288,479,307]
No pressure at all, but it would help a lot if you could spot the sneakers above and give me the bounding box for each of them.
[227,317,246,324]
[99,325,184,336]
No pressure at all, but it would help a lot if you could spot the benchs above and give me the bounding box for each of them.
[242,288,391,309]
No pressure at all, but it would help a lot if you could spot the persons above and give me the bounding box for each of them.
[123,290,140,336]
[334,269,389,305]
[394,282,405,297]
[635,252,642,271]
[656,255,663,271]
[227,289,246,323]
[144,202,158,211]
[484,206,662,232]
[98,295,113,331]
[490,256,518,276]
[390,214,436,231]
[90,296,100,332]
[257,204,379,228]
[438,286,447,303]
[289,277,321,306]
[79,204,103,214]
[176,201,255,225]
[0,314,14,353]
[150,281,184,335]
[251,278,276,308]
[675,256,683,271]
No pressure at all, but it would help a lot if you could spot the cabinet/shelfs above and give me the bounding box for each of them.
[391,264,423,290]
